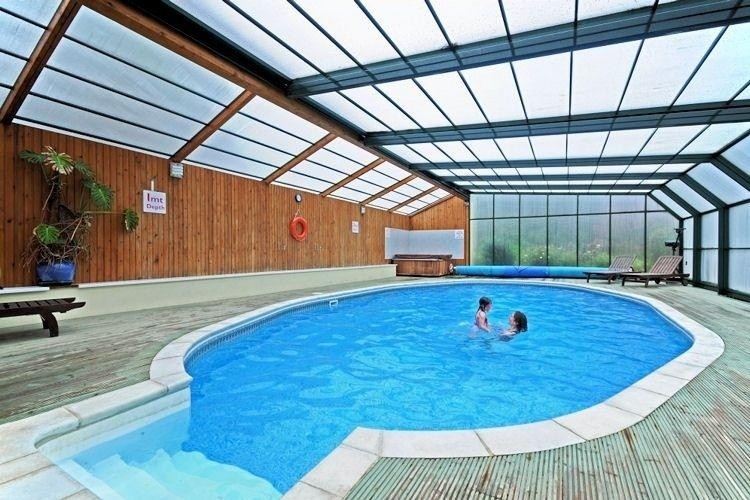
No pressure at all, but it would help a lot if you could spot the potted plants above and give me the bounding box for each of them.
[14,146,139,286]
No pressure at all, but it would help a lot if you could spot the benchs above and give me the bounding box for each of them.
[0,297,86,336]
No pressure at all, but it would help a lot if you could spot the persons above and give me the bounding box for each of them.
[497,310,528,339]
[473,294,492,334]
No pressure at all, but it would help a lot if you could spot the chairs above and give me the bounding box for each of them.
[582,254,690,287]
